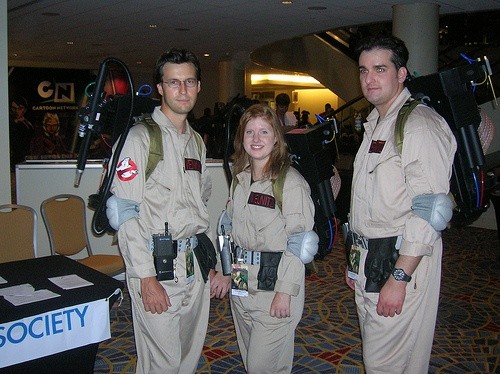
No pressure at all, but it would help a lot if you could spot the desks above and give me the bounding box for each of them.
[0,254,125,374]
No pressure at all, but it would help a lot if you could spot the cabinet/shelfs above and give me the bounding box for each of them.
[15,159,230,260]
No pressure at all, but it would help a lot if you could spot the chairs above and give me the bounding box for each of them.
[40,194,126,277]
[0,204,38,263]
